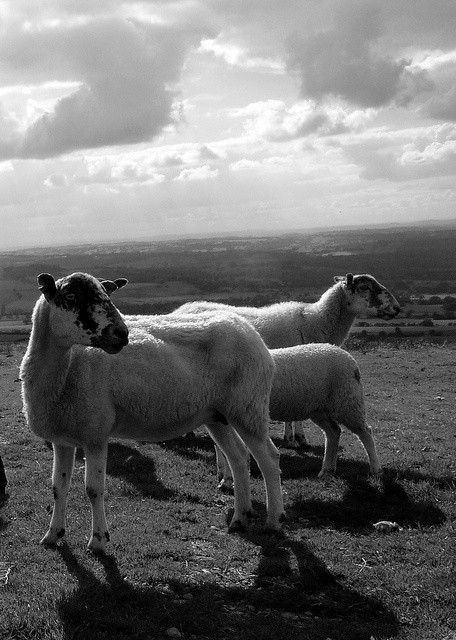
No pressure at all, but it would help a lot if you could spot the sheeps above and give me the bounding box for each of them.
[165,272,400,449]
[17,271,287,557]
[212,341,384,492]
